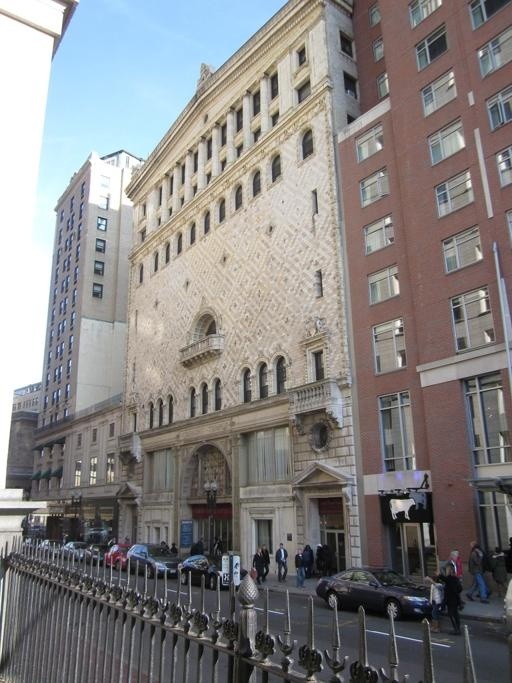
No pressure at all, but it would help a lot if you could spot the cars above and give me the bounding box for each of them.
[128,543,181,579]
[177,556,247,590]
[103,544,131,571]
[316,568,431,622]
[24,538,106,566]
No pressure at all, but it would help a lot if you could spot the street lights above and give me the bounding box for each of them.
[71,491,83,521]
[203,482,218,556]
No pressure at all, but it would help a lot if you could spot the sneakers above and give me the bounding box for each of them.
[258,575,312,589]
[430,591,493,635]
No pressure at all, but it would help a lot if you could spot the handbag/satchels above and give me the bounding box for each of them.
[250,567,258,581]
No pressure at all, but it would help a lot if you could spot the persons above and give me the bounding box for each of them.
[422,534,512,635]
[106,535,117,547]
[190,535,224,556]
[170,542,179,554]
[124,535,131,544]
[251,541,336,588]
[61,529,71,545]
[159,540,169,550]
